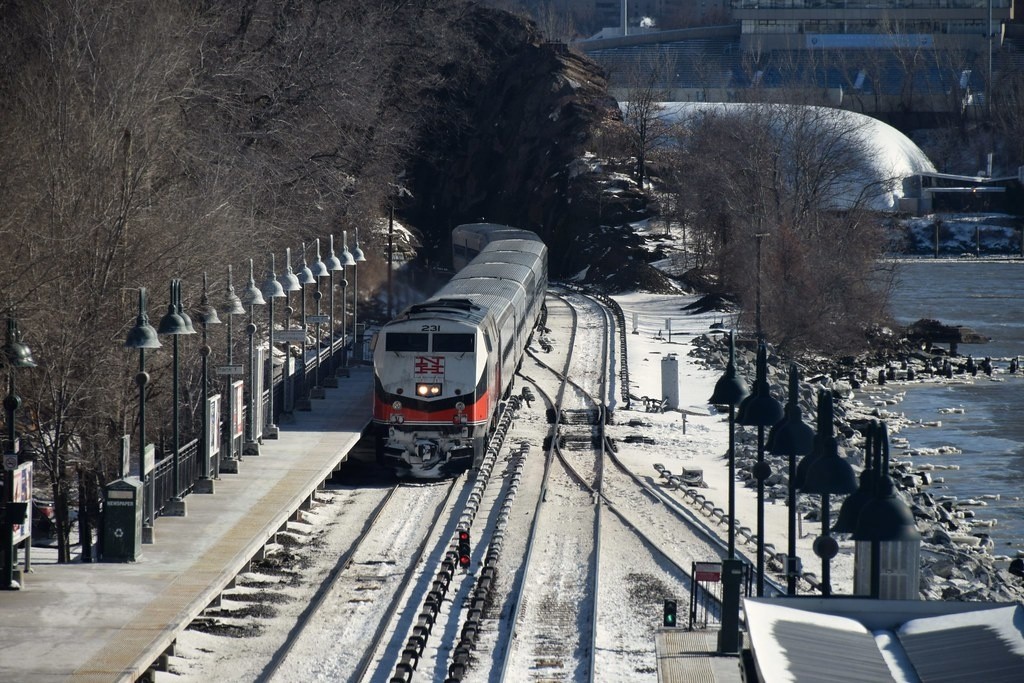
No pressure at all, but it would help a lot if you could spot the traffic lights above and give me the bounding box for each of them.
[664,598,677,627]
[459,529,471,568]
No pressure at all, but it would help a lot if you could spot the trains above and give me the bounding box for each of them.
[368,223,548,482]
